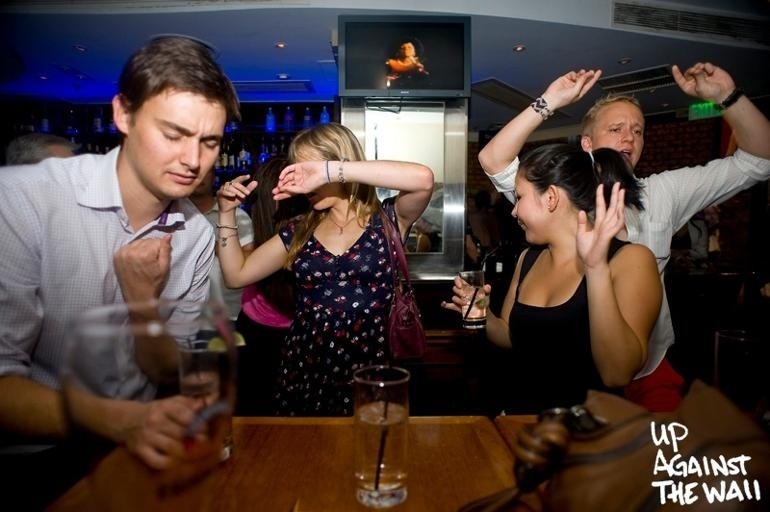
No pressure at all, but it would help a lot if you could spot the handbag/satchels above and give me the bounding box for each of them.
[453,377,770,512]
[381,196,431,373]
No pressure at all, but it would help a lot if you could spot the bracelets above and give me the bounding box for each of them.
[714,87,743,110]
[215,223,239,229]
[338,157,349,183]
[324,160,330,184]
[530,95,555,120]
[217,232,239,248]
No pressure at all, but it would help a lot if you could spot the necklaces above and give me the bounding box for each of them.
[329,215,356,234]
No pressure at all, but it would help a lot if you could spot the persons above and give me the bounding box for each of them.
[6,133,82,165]
[402,208,441,253]
[477,61,770,381]
[234,153,309,417]
[0,35,240,512]
[214,121,435,417]
[188,164,255,356]
[438,143,664,412]
[387,42,424,74]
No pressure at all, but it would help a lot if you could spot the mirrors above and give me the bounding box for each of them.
[364,99,445,256]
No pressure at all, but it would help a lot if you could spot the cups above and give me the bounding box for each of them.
[352,366,412,509]
[458,269,487,330]
[66,295,235,503]
[177,337,219,410]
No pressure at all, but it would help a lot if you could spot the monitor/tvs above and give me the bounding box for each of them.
[338,14,472,97]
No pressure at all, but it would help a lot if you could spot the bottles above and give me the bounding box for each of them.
[28,103,329,191]
